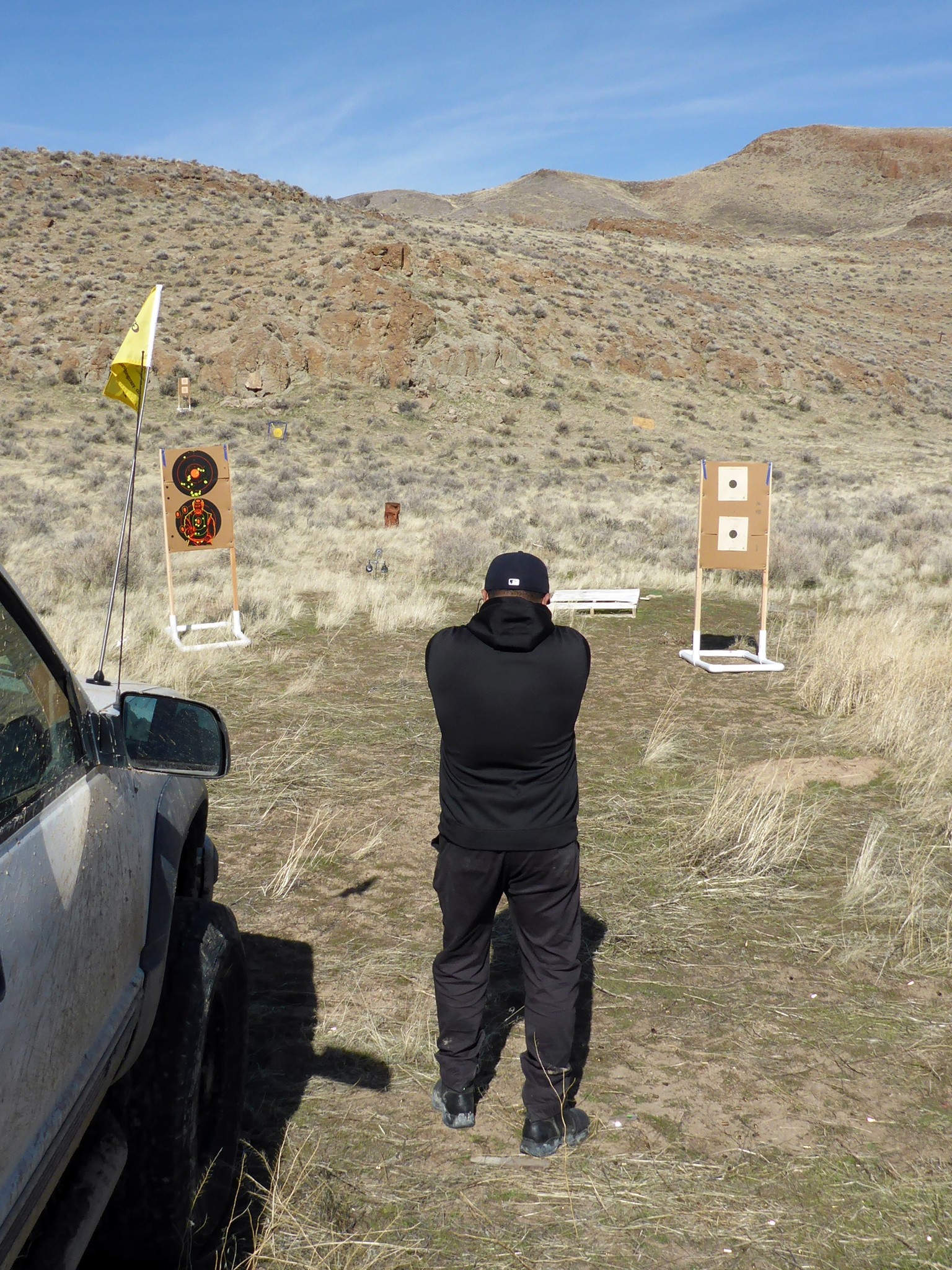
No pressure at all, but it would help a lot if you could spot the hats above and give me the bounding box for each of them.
[485,551,550,605]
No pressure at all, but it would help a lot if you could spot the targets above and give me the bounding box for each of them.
[174,497,222,546]
[717,465,748,502]
[172,450,219,498]
[717,515,749,552]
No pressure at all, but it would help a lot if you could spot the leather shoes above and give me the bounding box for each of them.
[432,1078,475,1129]
[519,1108,590,1157]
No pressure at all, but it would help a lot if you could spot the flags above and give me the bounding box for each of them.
[100,284,162,413]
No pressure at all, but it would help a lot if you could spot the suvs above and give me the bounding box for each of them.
[0,571,246,1270]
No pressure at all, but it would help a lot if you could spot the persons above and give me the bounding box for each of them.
[425,550,591,1159]
